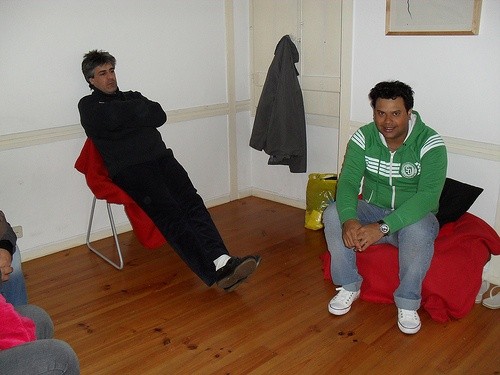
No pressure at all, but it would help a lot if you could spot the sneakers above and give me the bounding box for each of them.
[397,307,422,334]
[328,288,360,315]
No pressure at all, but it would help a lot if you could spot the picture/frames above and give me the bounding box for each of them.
[385,0,482,36]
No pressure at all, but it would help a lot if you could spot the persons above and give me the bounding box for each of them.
[0,211,81,375]
[322,79,448,334]
[77,49,263,292]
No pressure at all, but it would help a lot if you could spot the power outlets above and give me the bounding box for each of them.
[13,227,23,239]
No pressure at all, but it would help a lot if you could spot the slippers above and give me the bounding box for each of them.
[482,285,500,310]
[475,280,489,303]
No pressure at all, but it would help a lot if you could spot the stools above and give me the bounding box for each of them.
[320,209,500,324]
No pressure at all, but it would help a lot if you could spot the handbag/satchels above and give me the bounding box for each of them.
[305,173,338,231]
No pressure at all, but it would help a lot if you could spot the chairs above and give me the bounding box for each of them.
[74,137,167,270]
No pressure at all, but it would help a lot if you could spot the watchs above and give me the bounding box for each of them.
[377,219,390,237]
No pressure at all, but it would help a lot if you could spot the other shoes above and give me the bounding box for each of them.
[215,255,261,292]
